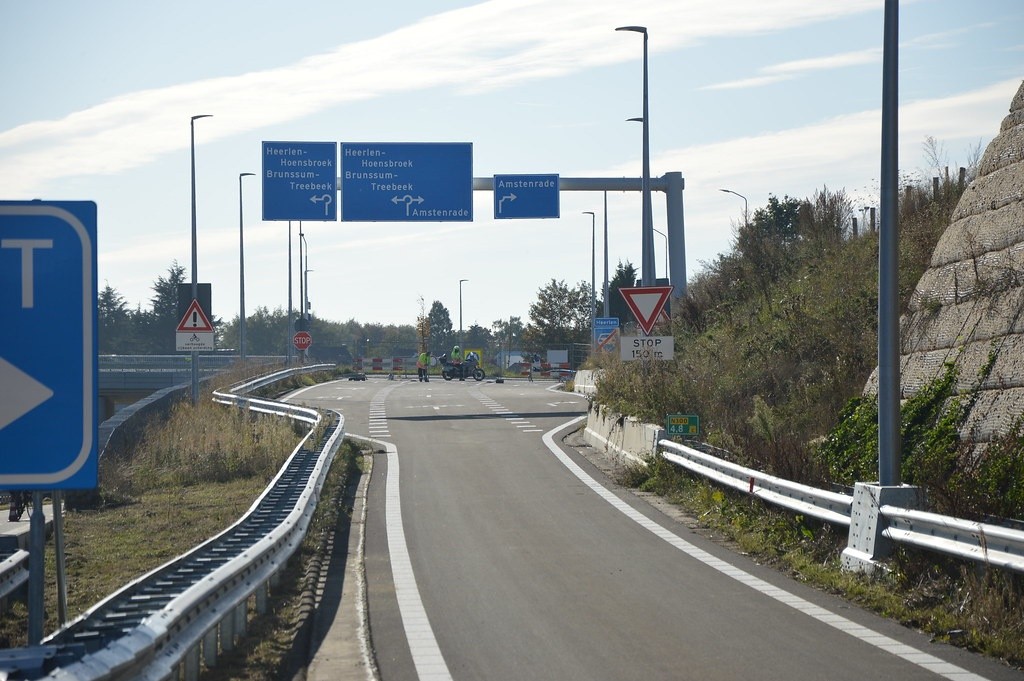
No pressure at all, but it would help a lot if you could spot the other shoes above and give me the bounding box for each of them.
[459,379,465,381]
[425,379,429,382]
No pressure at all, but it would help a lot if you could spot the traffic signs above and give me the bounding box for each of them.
[262,140,337,221]
[340,141,473,223]
[494,174,560,220]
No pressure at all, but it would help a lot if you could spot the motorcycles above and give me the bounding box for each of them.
[438,352,486,381]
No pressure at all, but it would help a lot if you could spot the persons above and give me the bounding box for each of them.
[451,346,465,381]
[416,351,431,382]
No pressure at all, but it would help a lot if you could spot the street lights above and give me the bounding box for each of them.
[582,212,595,357]
[305,270,316,363]
[191,113,215,409]
[240,172,258,374]
[722,189,748,227]
[615,25,657,286]
[459,280,469,347]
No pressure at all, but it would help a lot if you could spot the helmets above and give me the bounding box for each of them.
[453,346,459,352]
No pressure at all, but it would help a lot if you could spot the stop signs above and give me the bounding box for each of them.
[292,331,313,351]
